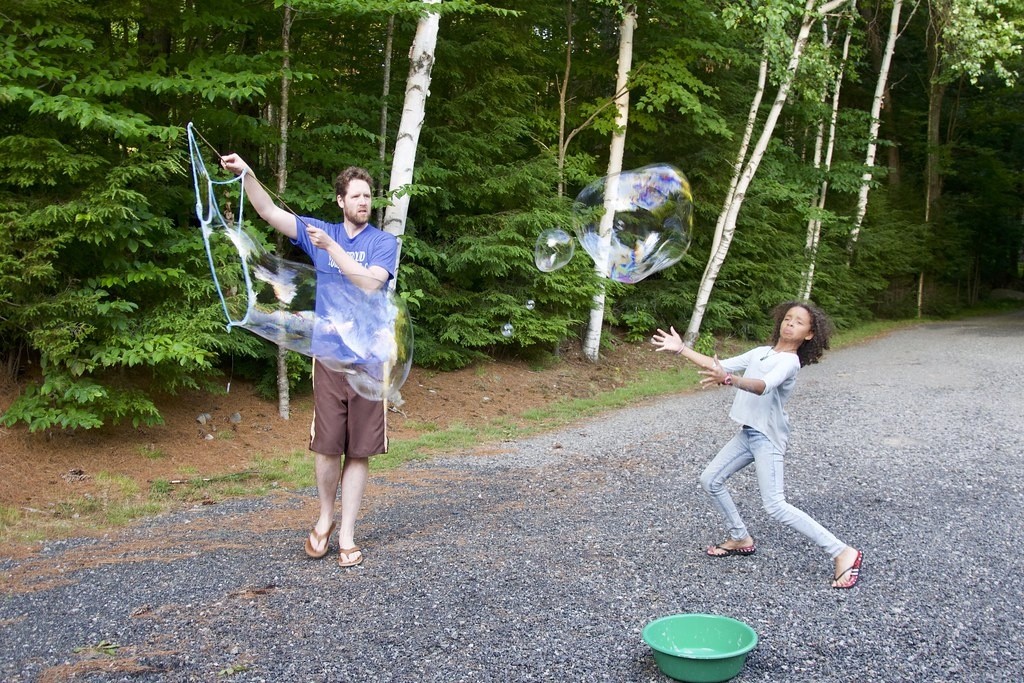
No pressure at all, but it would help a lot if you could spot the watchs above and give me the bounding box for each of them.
[721,372,733,385]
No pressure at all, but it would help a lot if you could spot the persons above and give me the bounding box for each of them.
[220,153,402,567]
[651,301,861,587]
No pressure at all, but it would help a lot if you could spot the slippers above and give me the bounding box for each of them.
[832,549,863,589]
[707,543,756,557]
[337,545,362,566]
[305,521,335,557]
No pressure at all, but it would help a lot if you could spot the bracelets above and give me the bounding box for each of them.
[674,344,684,355]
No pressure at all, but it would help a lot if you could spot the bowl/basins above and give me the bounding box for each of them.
[642,613,758,683]
